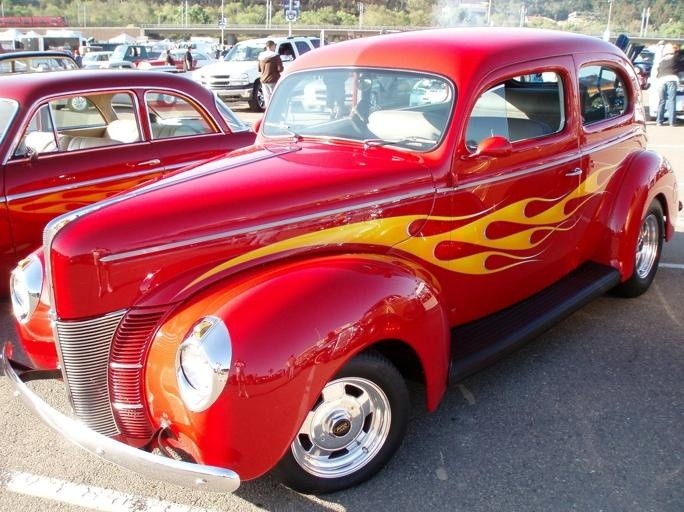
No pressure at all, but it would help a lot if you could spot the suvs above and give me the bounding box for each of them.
[190,33,330,114]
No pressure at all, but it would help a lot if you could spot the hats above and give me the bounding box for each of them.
[663,43,674,56]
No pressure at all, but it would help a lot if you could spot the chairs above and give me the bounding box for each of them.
[367,86,586,148]
[25,118,202,154]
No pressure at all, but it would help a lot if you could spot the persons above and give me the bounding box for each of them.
[210,45,219,60]
[256,40,283,109]
[164,50,174,66]
[220,46,228,58]
[184,46,192,71]
[654,44,682,127]
[320,70,349,122]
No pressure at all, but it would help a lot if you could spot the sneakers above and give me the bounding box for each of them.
[657,123,679,126]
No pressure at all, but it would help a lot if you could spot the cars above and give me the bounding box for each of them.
[0,50,94,113]
[0,25,683,497]
[80,42,214,108]
[513,33,684,120]
[409,77,452,107]
[0,67,259,303]
[303,75,415,113]
